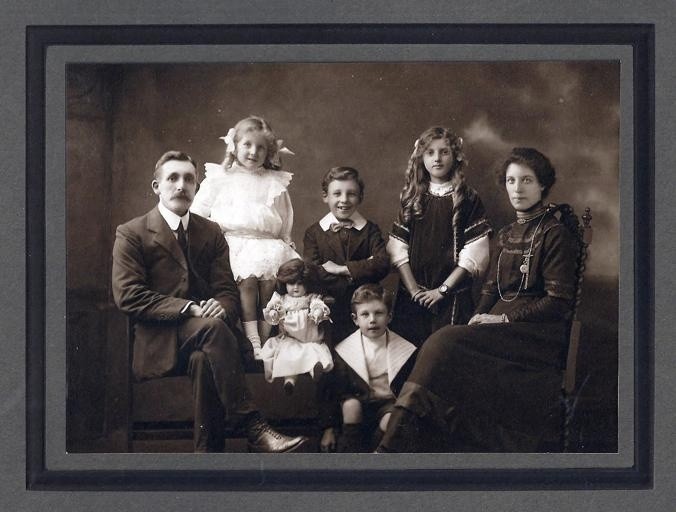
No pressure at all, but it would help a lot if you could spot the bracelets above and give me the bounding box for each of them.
[411,289,424,301]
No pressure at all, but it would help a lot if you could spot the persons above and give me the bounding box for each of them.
[190,115,303,362]
[260,258,334,398]
[112,151,315,454]
[302,166,390,351]
[373,147,580,455]
[385,126,493,355]
[319,283,418,454]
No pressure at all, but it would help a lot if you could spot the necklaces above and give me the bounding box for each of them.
[496,210,547,303]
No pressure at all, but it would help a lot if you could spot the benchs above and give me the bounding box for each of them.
[122,304,335,449]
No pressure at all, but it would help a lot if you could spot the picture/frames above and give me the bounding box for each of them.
[27,20,655,490]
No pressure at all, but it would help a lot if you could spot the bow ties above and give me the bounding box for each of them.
[330,221,354,233]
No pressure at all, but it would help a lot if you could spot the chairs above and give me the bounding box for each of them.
[452,205,593,449]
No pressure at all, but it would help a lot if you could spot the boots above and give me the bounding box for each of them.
[245,411,311,453]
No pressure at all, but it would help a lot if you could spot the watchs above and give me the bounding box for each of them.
[438,284,449,296]
